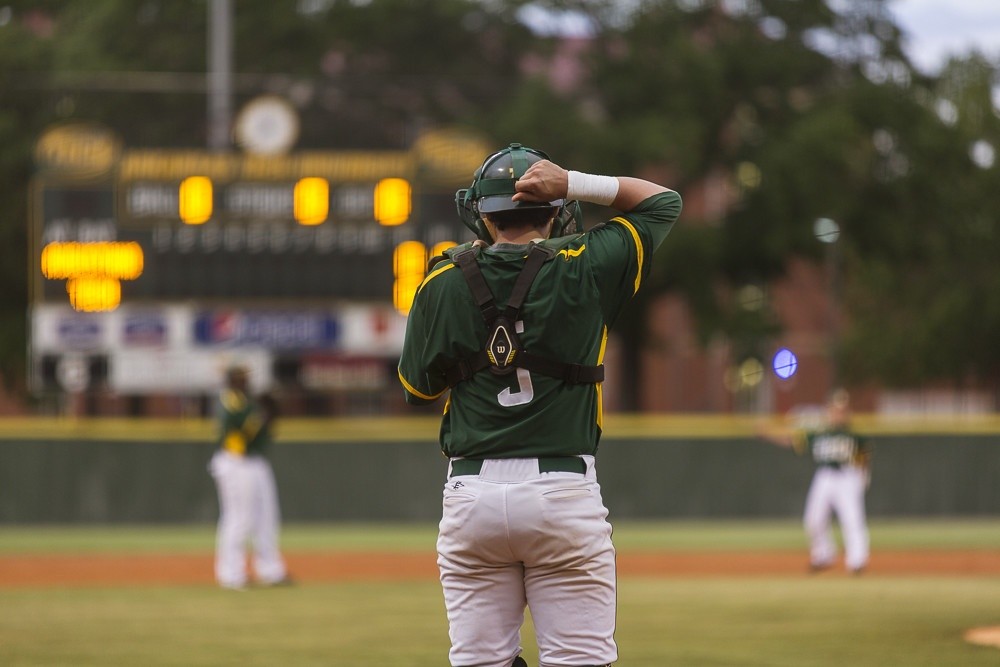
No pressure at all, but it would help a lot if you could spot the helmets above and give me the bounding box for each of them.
[473,142,564,218]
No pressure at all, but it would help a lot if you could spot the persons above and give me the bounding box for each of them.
[209,370,294,589]
[754,402,873,572]
[397,142,683,667]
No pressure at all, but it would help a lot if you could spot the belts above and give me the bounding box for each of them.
[447,456,588,479]
